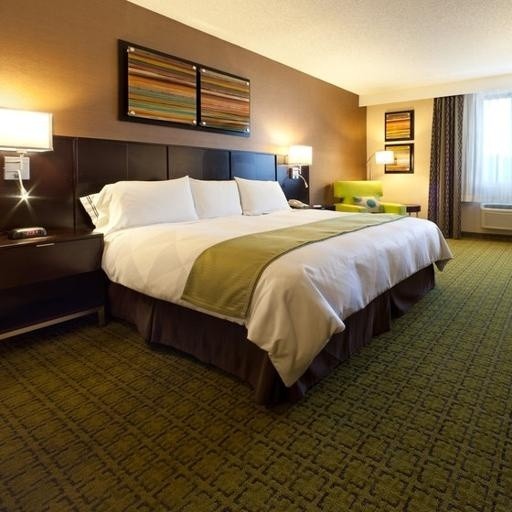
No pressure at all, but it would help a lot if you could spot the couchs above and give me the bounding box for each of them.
[334,180,406,215]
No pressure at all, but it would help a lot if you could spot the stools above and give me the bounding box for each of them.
[407,205,420,219]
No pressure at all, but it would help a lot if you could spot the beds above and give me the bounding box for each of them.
[71,136,453,404]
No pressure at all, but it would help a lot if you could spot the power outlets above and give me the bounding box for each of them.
[292,167,299,179]
[4,156,30,180]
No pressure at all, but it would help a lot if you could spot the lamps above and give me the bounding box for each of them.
[358,150,394,165]
[288,144,313,188]
[0,109,53,193]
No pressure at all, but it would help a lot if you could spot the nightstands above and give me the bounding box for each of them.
[0,226,105,340]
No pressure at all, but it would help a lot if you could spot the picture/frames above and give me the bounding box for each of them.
[384,110,413,142]
[385,144,413,174]
[118,39,199,131]
[199,64,250,138]
